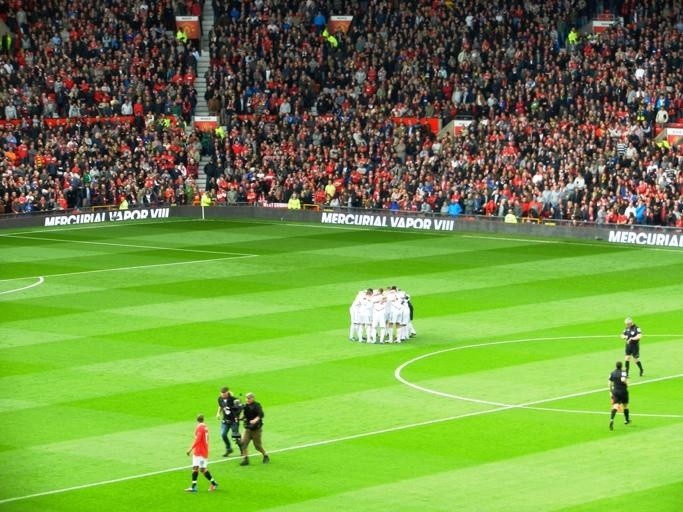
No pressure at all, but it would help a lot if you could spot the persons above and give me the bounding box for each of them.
[349,286,416,344]
[215,387,241,457]
[608,361,632,430]
[622,317,644,376]
[1,0,683,232]
[238,392,271,466]
[184,414,219,493]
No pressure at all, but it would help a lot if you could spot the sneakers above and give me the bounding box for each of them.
[240,461,249,465]
[185,488,197,492]
[223,448,232,457]
[262,456,269,463]
[209,483,217,492]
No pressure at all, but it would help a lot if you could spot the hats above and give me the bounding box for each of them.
[246,393,254,399]
